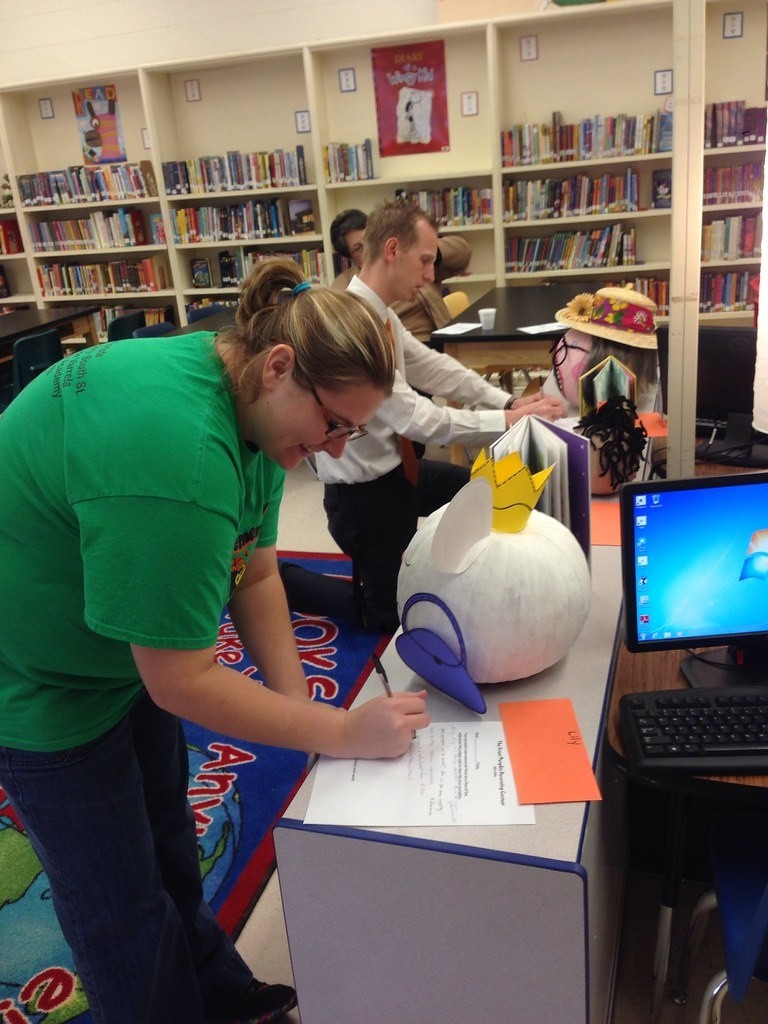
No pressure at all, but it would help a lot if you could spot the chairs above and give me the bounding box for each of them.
[132,322,174,339]
[107,310,146,342]
[6,329,64,402]
[188,305,231,324]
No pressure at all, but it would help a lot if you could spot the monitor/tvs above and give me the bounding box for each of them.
[619,471,768,688]
[657,324,768,468]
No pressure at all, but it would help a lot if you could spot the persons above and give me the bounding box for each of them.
[0,252,429,1024]
[330,208,472,458]
[276,200,565,631]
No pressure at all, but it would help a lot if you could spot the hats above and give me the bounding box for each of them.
[553,286,658,351]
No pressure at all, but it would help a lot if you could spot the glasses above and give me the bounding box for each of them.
[348,236,366,259]
[306,379,369,443]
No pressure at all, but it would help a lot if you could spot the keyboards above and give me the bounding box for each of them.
[618,685,768,777]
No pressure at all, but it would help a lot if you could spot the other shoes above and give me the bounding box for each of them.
[213,977,300,1024]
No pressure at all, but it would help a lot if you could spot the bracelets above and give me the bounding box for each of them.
[506,397,516,409]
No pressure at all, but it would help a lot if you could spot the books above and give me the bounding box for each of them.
[185,298,241,325]
[164,145,307,195]
[323,139,374,182]
[37,258,164,296]
[22,163,156,206]
[501,111,672,167]
[505,224,636,272]
[29,207,166,253]
[539,274,668,315]
[191,246,325,288]
[94,303,172,337]
[170,199,316,243]
[0,219,24,254]
[333,254,354,278]
[488,414,590,565]
[503,168,671,224]
[700,100,768,314]
[394,186,492,226]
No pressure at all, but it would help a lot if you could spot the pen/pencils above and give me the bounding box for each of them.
[371,652,394,696]
[538,375,544,395]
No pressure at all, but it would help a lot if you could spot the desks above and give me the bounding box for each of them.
[160,305,239,337]
[0,305,102,365]
[431,280,609,468]
[607,429,768,790]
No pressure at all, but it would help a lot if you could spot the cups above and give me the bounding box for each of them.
[478,308,496,330]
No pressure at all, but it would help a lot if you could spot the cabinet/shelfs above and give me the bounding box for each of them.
[689,0,768,328]
[272,381,661,1024]
[0,0,687,481]
